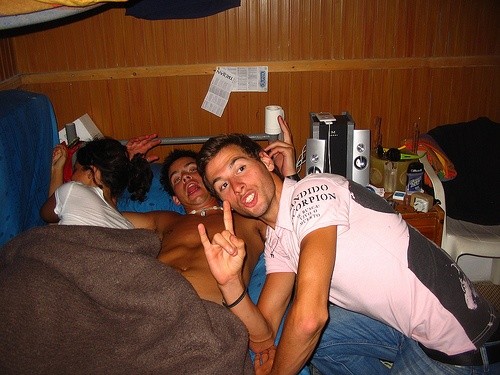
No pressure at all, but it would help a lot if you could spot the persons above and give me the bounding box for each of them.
[112,116,301,310]
[193,133,500,375]
[40,136,154,230]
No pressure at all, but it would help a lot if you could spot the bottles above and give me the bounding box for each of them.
[406,162,424,195]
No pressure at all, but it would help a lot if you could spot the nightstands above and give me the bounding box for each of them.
[393,201,444,246]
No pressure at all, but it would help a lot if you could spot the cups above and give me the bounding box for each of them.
[384,161,397,193]
[264,105,285,135]
[370,155,387,187]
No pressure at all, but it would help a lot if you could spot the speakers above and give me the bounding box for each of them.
[306,112,370,187]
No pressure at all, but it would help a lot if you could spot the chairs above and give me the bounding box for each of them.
[416,147,500,264]
[455,254,500,349]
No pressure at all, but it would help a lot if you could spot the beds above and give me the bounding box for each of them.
[68,130,448,375]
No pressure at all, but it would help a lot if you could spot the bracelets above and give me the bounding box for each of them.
[287,173,301,182]
[222,287,247,309]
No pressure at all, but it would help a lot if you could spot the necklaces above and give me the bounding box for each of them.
[188,205,224,217]
[268,227,283,258]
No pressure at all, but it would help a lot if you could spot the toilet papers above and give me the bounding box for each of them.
[264,105,284,136]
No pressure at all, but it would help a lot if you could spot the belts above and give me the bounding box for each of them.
[418,341,500,365]
